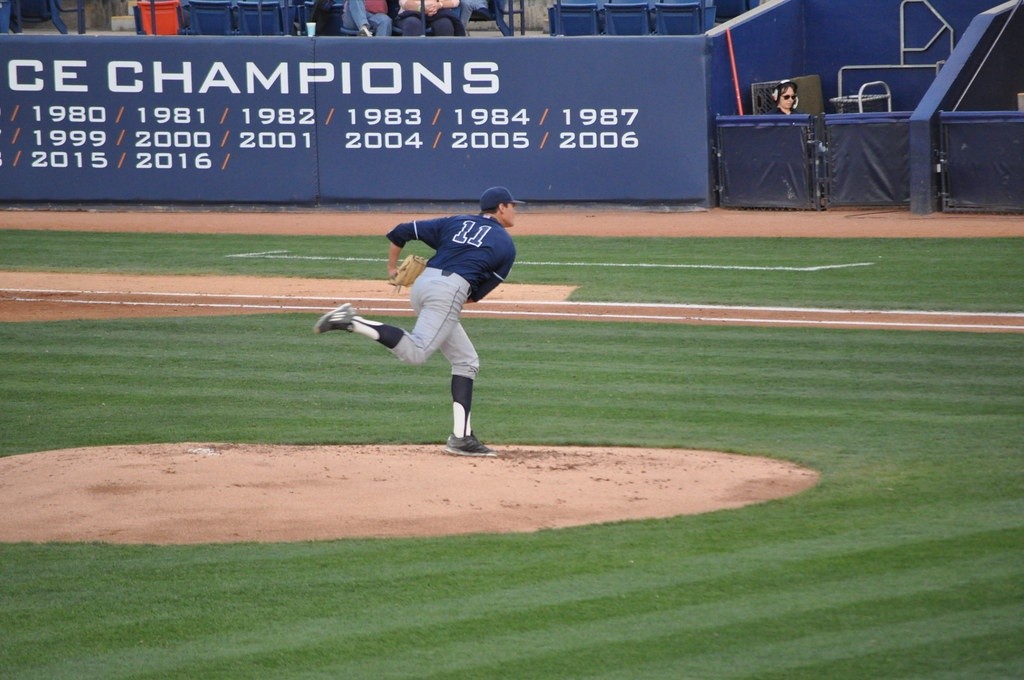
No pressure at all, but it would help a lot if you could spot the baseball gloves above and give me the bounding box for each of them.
[388,254,428,287]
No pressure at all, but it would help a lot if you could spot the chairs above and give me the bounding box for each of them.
[0,0,760,35]
[750,80,779,115]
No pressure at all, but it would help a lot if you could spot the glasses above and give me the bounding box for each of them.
[782,95,797,100]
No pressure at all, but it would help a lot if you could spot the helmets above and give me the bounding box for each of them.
[772,80,798,90]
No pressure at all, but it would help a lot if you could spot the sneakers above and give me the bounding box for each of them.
[444,433,497,457]
[314,303,359,334]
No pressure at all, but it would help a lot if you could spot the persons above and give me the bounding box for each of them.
[766,82,800,114]
[314,187,525,456]
[342,0,505,36]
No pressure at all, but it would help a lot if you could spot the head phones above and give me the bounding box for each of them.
[771,79,798,110]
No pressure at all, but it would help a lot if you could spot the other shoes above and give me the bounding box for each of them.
[359,26,372,36]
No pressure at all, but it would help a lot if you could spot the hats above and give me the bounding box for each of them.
[480,186,526,210]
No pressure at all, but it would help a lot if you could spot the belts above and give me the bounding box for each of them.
[442,270,472,300]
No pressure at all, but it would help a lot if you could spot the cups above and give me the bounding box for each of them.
[306,23,316,37]
